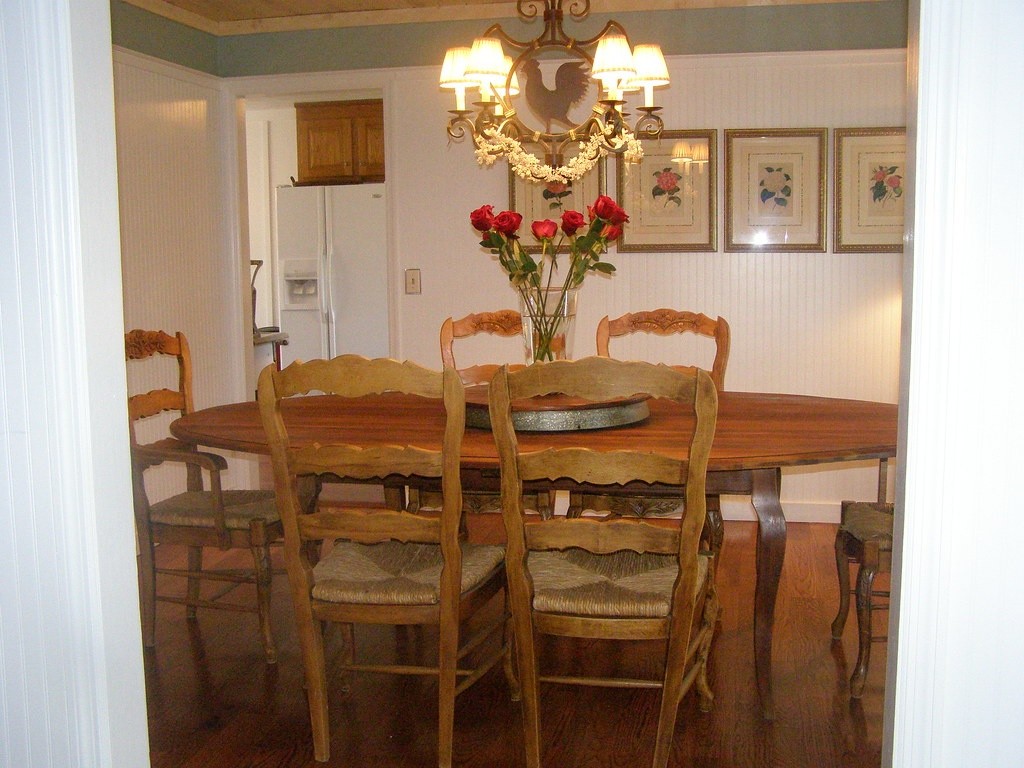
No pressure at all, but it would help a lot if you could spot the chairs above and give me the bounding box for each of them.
[120,309,897,768]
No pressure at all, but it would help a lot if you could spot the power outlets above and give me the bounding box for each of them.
[405,269,422,295]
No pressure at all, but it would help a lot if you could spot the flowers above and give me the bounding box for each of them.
[467,194,630,362]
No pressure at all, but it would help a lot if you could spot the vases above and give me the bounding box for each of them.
[517,286,577,373]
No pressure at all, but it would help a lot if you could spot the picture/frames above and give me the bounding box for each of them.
[723,127,828,254]
[509,132,609,256]
[613,128,719,255]
[833,126,906,254]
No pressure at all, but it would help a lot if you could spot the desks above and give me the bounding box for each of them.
[168,390,896,719]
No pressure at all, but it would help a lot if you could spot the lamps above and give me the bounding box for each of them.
[437,0,671,186]
[671,140,709,175]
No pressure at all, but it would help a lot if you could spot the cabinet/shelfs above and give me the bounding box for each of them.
[293,99,385,187]
